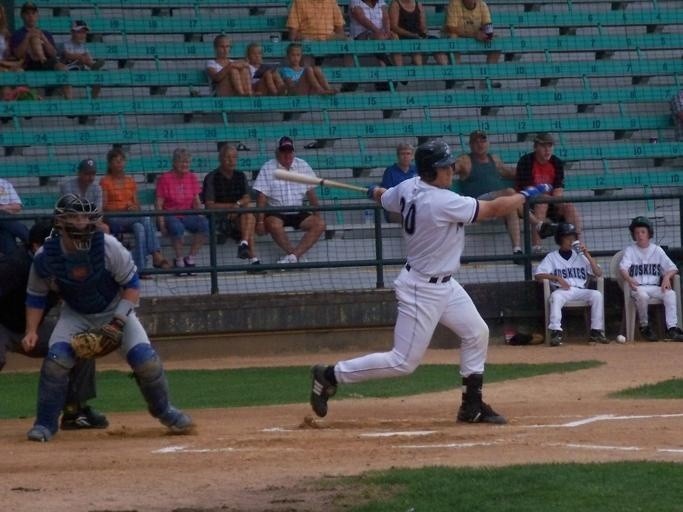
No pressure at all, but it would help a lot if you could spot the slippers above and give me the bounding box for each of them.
[151,258,170,270]
[138,275,155,281]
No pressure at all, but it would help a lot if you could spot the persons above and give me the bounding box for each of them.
[252,134,323,263]
[533,221,612,346]
[21,193,197,442]
[512,131,584,258]
[199,142,267,275]
[453,131,559,264]
[381,141,420,223]
[309,141,553,423]
[0,0,104,122]
[152,144,209,278]
[101,146,171,280]
[204,0,501,97]
[618,213,683,342]
[1,158,110,430]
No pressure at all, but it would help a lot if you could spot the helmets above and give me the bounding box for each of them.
[51,193,96,241]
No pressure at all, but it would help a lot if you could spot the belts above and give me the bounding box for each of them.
[404,261,452,284]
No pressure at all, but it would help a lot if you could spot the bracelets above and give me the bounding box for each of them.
[236,200,241,206]
[256,219,264,224]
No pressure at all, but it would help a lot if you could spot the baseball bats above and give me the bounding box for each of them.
[273,170,369,195]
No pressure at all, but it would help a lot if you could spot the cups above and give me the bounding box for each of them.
[571,240,584,255]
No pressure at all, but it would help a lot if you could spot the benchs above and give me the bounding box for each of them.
[1,0,682,293]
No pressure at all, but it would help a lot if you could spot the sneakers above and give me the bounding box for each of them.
[584,330,611,344]
[509,250,526,265]
[311,362,336,418]
[637,326,658,342]
[25,420,59,442]
[157,403,192,435]
[275,254,308,271]
[245,260,269,275]
[536,220,561,240]
[174,256,197,277]
[455,402,507,424]
[237,242,250,259]
[662,327,683,343]
[60,405,109,429]
[549,331,563,346]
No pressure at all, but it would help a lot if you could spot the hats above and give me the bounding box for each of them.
[70,19,91,32]
[414,139,458,183]
[275,136,296,152]
[20,2,37,12]
[469,129,487,150]
[555,223,580,245]
[533,133,554,144]
[628,215,653,242]
[77,158,99,174]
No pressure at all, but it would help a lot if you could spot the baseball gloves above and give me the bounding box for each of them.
[72,319,123,358]
[507,329,543,345]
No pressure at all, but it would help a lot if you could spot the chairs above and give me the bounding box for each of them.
[541,272,607,345]
[610,246,681,342]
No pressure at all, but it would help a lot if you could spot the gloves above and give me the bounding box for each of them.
[366,184,380,200]
[518,182,554,200]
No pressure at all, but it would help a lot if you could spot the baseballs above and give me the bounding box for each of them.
[617,334,626,342]
